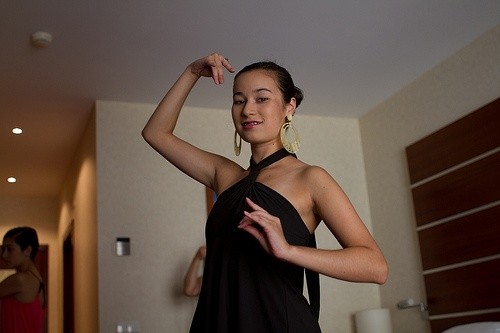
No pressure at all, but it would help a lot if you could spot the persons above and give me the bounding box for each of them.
[141,54,388,333]
[0,227,47,333]
[184,245,206,297]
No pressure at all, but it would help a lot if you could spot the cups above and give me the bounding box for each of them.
[116,321,141,333]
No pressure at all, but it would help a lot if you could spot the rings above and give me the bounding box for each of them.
[222,58,228,63]
